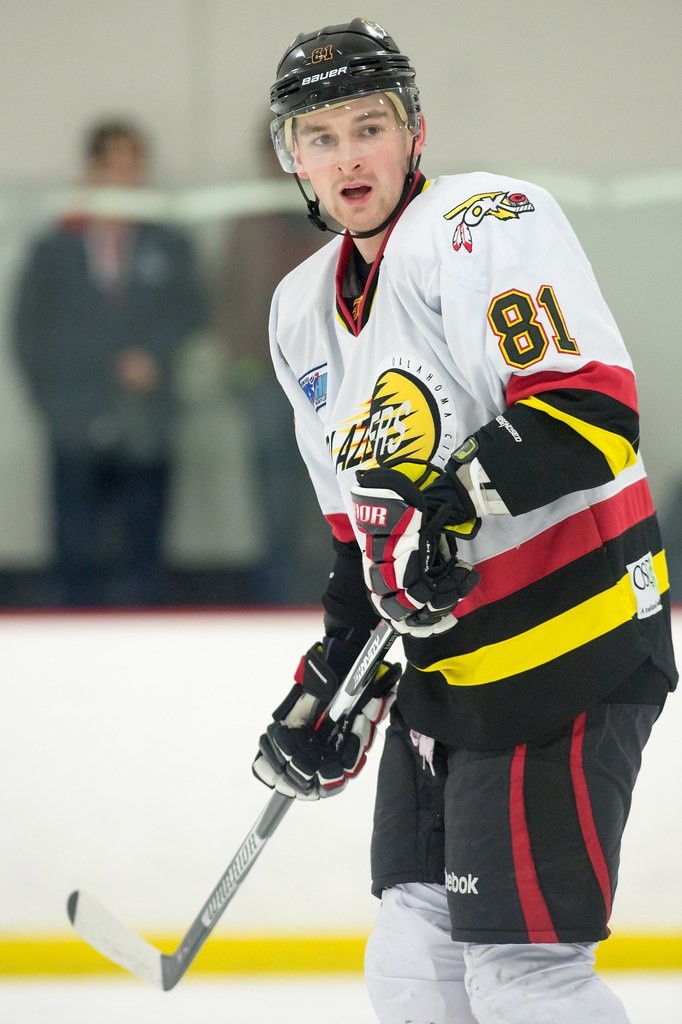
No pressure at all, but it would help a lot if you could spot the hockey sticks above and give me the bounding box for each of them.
[64,618,398,994]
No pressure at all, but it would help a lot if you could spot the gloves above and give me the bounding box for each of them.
[349,469,482,641]
[251,645,398,800]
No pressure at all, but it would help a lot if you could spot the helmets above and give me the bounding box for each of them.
[269,19,423,142]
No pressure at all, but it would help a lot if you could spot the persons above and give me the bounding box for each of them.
[251,17,679,1024]
[211,115,342,592]
[18,119,218,592]
